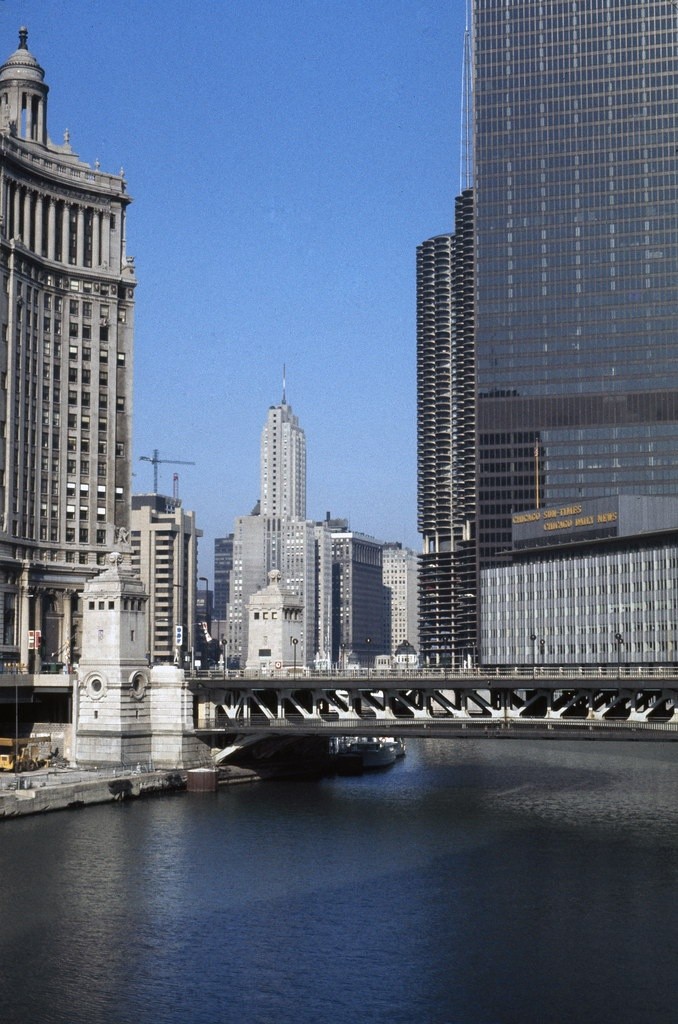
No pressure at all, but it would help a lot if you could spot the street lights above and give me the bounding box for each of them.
[341,643,345,677]
[292,639,298,679]
[405,642,409,666]
[530,634,536,663]
[540,639,545,662]
[616,633,623,662]
[222,639,227,680]
[366,638,370,677]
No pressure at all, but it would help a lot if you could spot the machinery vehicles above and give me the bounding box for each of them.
[0,736,52,773]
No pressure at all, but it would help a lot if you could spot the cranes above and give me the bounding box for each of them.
[137,449,196,495]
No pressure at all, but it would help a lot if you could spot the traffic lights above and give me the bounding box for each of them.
[4,662,30,776]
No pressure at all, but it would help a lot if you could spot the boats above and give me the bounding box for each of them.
[328,687,407,769]
